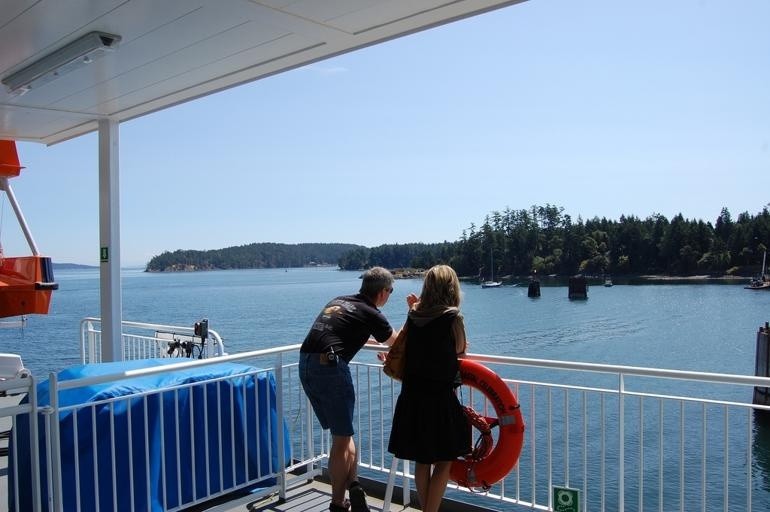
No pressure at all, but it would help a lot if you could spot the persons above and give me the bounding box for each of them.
[299,266,420,512]
[383,264,472,512]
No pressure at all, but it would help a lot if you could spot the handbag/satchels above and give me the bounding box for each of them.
[382,318,408,381]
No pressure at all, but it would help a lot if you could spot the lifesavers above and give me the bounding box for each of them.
[448,358,524,487]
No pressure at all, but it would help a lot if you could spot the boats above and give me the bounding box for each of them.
[604,280,612,287]
[482,280,502,288]
[744,251,770,289]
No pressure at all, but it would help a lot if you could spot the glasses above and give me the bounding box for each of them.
[386,286,394,294]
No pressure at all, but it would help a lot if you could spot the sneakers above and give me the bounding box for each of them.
[349,480,371,512]
[329,499,351,511]
